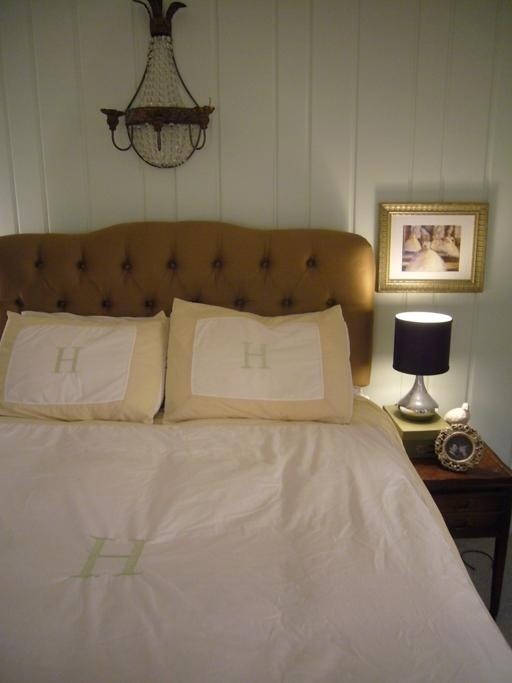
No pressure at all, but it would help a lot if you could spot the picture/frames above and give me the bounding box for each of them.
[376,202,490,294]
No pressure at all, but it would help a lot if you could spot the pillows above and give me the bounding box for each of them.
[165,297,354,425]
[2,310,169,426]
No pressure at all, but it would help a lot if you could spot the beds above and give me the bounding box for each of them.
[0,218,512,683]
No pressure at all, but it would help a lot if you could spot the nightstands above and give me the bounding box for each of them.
[412,449,512,622]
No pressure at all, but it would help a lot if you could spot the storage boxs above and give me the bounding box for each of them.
[383,402,452,460]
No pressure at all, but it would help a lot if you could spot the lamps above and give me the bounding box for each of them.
[99,1,216,173]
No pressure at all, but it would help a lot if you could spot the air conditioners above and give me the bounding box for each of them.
[392,310,452,411]
